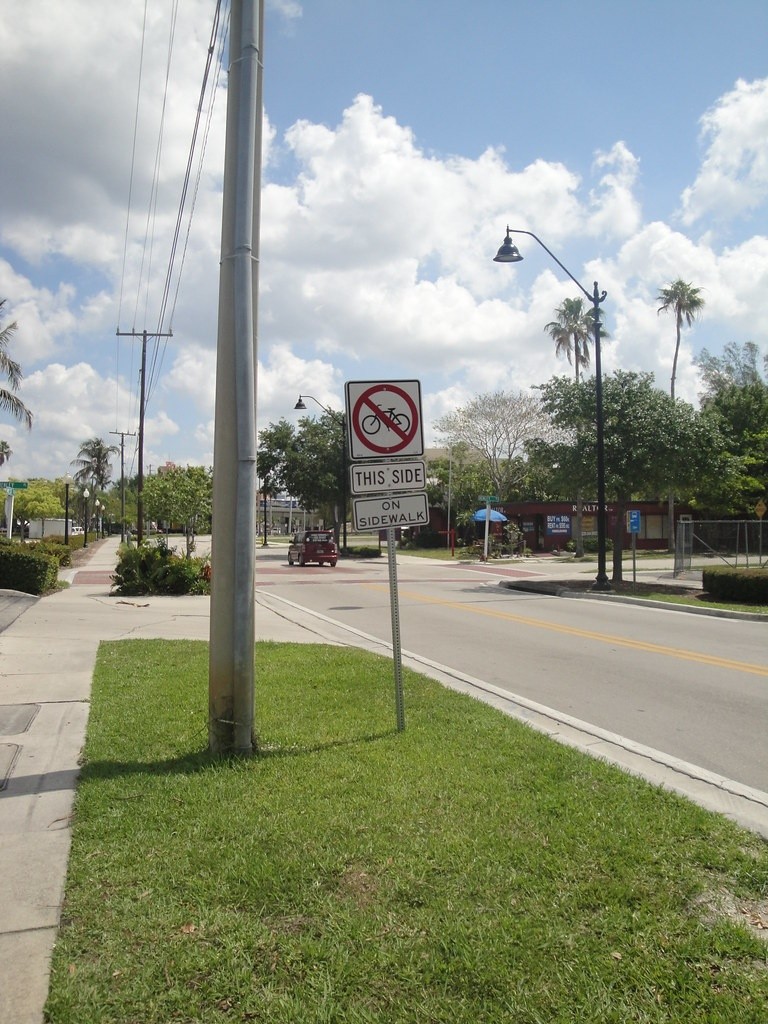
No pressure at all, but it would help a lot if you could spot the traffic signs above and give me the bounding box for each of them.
[478,495,499,502]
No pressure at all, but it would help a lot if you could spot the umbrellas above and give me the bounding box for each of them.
[470,509,507,521]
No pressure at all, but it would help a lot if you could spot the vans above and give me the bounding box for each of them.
[288,530,338,567]
[72,527,84,535]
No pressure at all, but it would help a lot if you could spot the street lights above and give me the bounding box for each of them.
[83,488,89,548]
[294,394,349,558]
[101,504,105,539]
[63,472,72,545]
[493,225,615,594]
[95,499,100,541]
